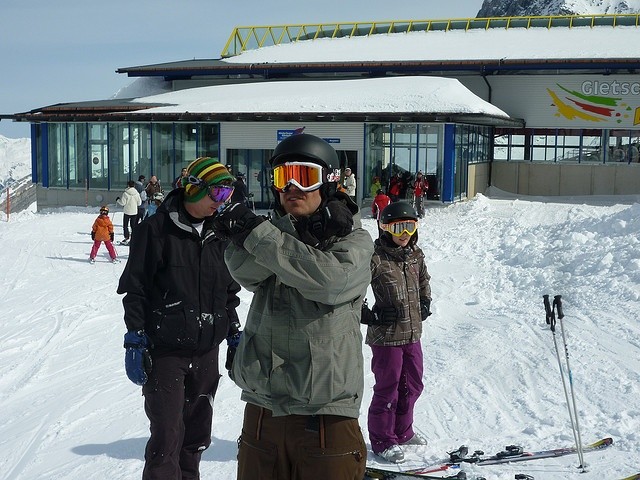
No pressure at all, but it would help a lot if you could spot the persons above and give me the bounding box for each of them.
[341,168,356,202]
[231,171,254,205]
[117,157,242,479]
[361,202,432,464]
[88,206,122,265]
[370,169,428,238]
[172,167,186,188]
[211,132,375,478]
[116,175,165,246]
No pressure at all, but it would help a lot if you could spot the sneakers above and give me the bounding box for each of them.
[378,445,404,463]
[90,258,95,263]
[113,258,121,263]
[120,239,130,245]
[399,433,426,445]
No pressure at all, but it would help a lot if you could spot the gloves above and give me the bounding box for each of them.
[420,296,429,321]
[92,231,96,239]
[295,200,354,248]
[116,197,119,201]
[226,331,243,379]
[110,233,114,241]
[361,305,398,326]
[124,331,152,385]
[211,202,265,246]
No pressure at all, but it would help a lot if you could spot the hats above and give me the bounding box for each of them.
[181,157,233,202]
[153,192,164,203]
[100,206,109,215]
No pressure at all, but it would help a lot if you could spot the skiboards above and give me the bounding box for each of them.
[88,258,119,266]
[446,438,613,466]
[361,465,471,480]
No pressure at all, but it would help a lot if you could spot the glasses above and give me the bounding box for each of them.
[271,162,341,193]
[189,176,235,204]
[379,220,416,237]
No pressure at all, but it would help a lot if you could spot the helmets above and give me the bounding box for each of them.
[380,202,417,224]
[269,134,340,173]
